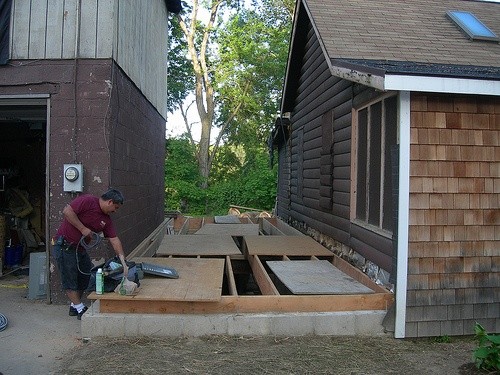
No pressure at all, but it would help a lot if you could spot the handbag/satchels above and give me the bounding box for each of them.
[85,258,139,291]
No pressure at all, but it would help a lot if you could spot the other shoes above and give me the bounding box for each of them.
[69,306,78,316]
[77,306,88,319]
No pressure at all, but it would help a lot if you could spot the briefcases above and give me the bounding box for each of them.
[136,262,178,280]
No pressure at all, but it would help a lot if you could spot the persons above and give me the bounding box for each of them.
[50,189,129,320]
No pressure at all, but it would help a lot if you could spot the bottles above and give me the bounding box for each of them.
[96,268,104,295]
